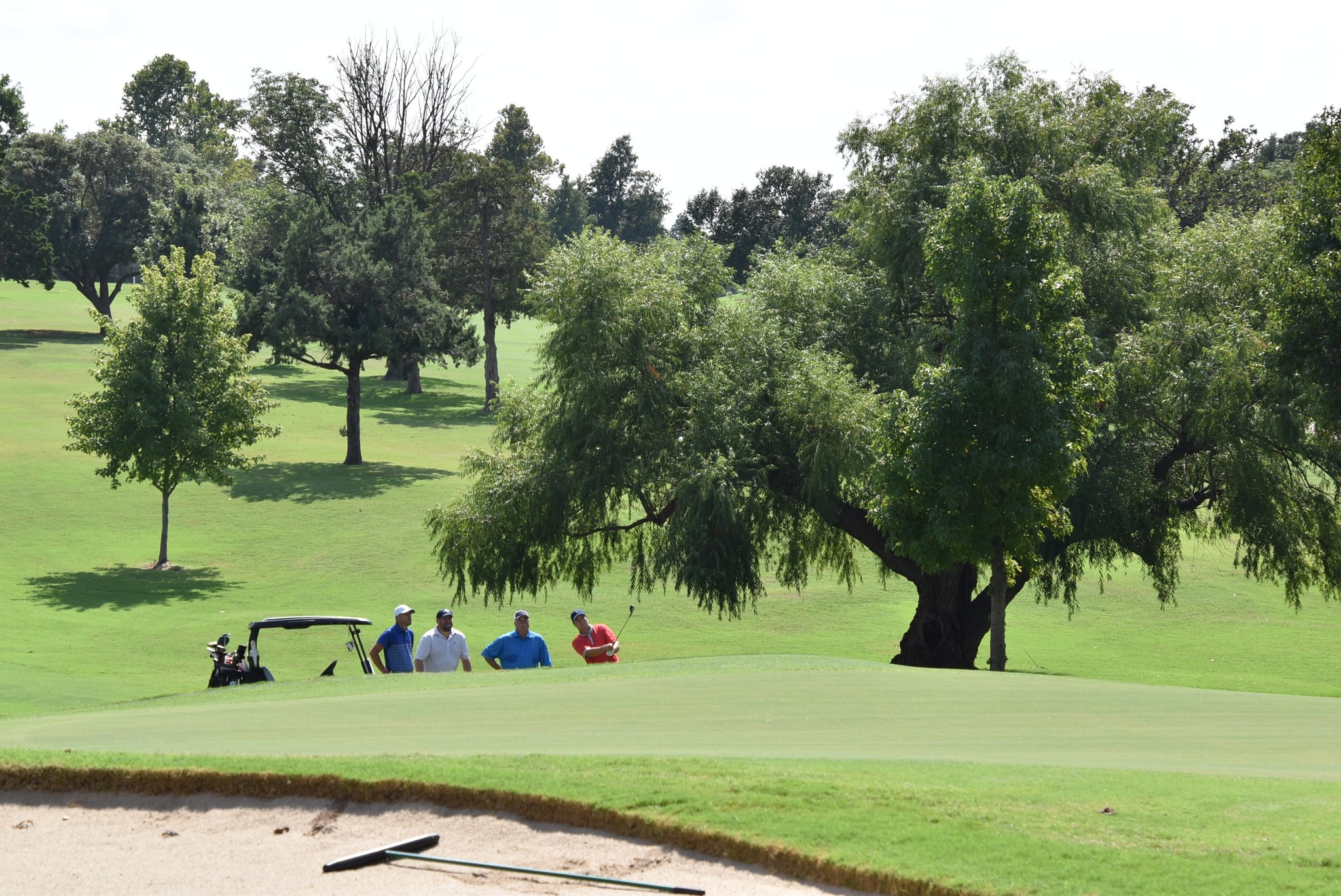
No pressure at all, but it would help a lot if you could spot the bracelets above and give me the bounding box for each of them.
[382,668,386,673]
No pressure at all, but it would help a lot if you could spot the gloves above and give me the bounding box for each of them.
[606,650,614,656]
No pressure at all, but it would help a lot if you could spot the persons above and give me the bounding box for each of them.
[414,609,471,673]
[570,609,620,664]
[481,610,553,670]
[369,604,414,674]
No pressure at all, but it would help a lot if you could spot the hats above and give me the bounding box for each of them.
[436,609,453,618]
[394,604,415,616]
[571,609,587,621]
[513,610,529,620]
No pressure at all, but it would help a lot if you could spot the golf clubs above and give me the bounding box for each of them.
[611,604,635,648]
[206,633,232,664]
